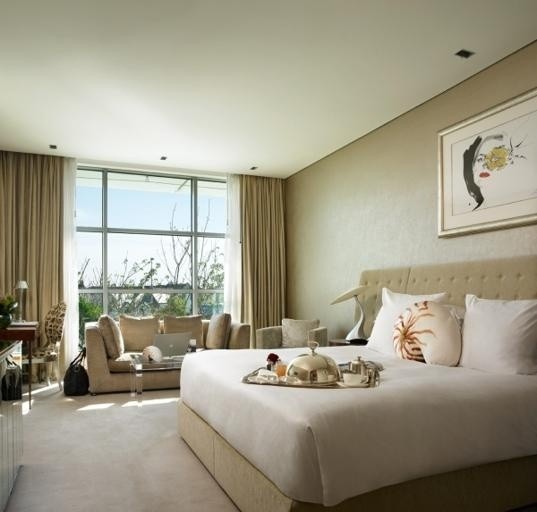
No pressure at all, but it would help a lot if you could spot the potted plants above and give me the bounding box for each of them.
[0,293,17,328]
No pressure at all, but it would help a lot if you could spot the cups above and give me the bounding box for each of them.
[366,369,376,387]
[184,338,196,352]
[342,373,368,384]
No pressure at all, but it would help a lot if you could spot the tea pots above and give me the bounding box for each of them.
[348,356,367,374]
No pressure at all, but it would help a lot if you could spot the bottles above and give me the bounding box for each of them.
[157,320,165,334]
[265,358,285,376]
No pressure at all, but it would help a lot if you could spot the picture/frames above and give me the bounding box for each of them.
[436,87,536,237]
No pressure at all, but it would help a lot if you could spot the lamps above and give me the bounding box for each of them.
[14,280,28,320]
[326,284,370,340]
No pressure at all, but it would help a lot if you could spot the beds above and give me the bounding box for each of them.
[172,255,537,512]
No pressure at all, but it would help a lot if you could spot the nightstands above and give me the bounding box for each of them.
[328,339,351,346]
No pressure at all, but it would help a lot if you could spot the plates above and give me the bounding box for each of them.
[335,381,368,388]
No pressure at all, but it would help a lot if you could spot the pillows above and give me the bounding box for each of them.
[366,286,448,354]
[458,293,537,374]
[119,314,161,352]
[205,313,230,349]
[98,315,125,358]
[163,314,204,348]
[394,301,462,368]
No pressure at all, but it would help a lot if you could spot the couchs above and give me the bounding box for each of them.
[255,317,328,348]
[83,312,251,395]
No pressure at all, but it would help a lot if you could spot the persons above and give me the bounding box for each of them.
[471,134,505,187]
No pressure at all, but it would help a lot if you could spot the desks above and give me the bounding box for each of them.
[0,323,36,411]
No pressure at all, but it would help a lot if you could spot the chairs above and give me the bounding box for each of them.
[20,302,67,391]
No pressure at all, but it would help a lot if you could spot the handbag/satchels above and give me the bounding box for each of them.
[64,347,90,396]
[2,354,22,401]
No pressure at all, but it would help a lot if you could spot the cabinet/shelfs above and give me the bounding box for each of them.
[0,339,21,511]
